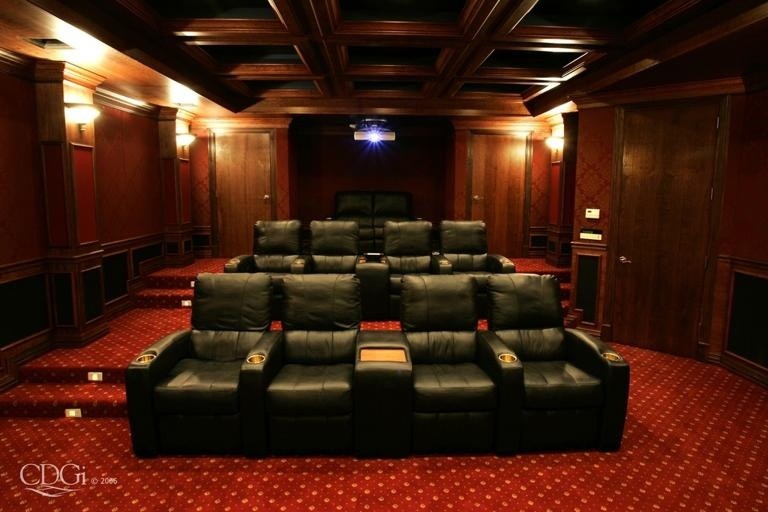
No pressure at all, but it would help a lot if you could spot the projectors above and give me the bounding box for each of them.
[354,125,396,142]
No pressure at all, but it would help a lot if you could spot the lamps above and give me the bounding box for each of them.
[352,118,397,144]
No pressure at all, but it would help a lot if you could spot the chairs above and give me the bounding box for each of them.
[127,190,631,459]
